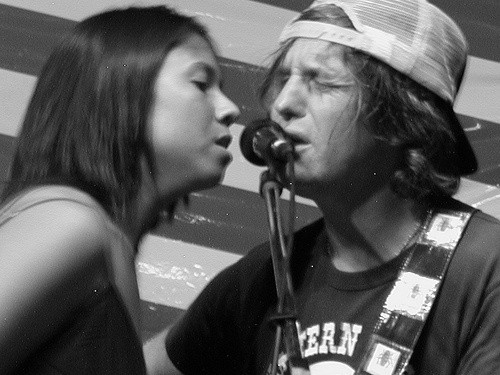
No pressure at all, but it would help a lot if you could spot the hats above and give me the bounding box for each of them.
[279,0,468,105]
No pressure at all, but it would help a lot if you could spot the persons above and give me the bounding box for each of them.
[0,5,242,375]
[142,1,500,375]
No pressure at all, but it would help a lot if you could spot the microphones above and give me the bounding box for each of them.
[240,119,293,166]
